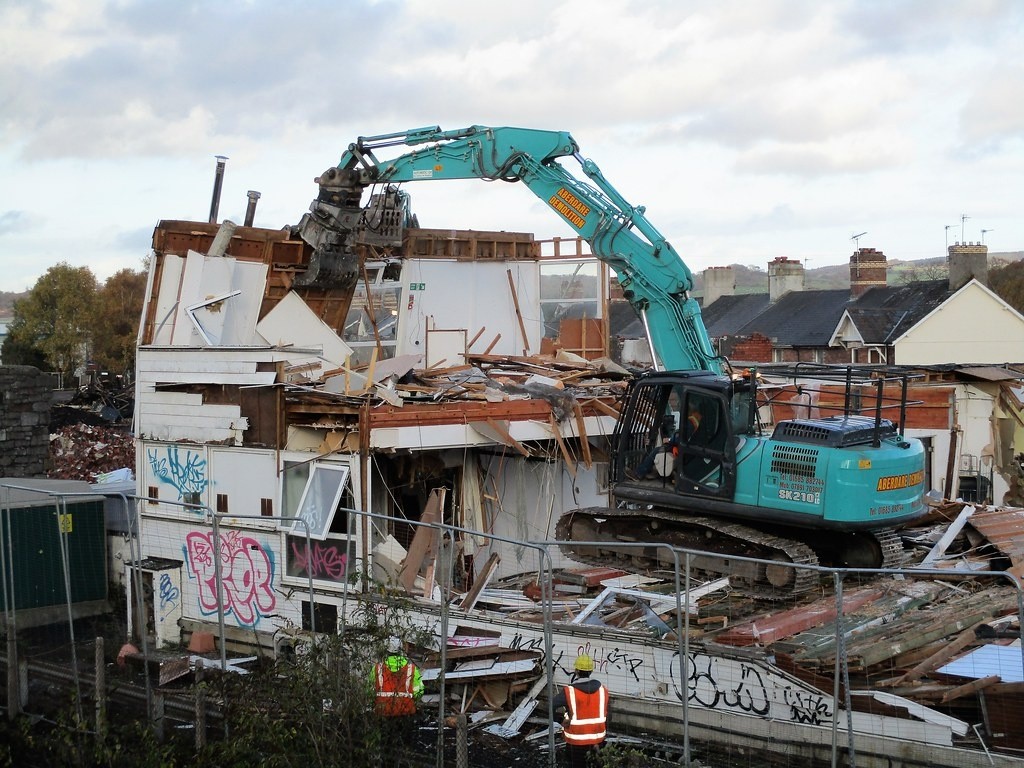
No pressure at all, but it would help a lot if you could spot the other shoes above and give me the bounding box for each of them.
[623,466,640,482]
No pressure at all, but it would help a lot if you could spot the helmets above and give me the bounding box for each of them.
[742,369,750,376]
[574,655,593,671]
[385,635,402,653]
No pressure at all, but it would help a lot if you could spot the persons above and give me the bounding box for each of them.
[367,636,424,768]
[553,654,609,768]
[623,389,707,483]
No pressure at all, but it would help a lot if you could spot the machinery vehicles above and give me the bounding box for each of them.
[280,125,928,600]
